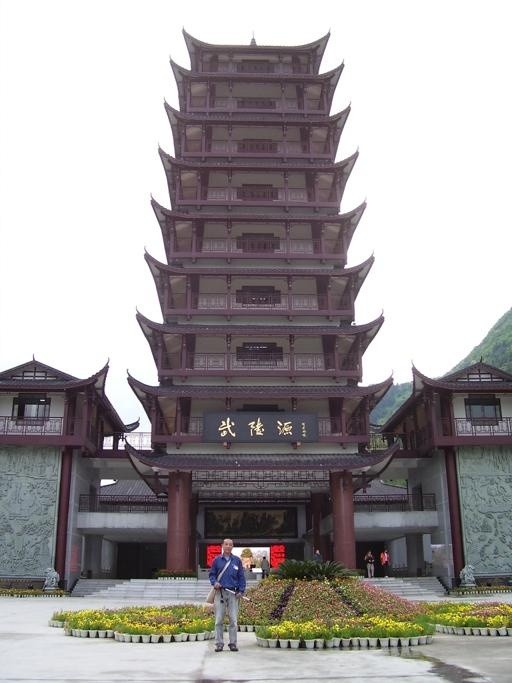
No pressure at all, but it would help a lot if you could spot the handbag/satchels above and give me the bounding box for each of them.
[207,588,216,604]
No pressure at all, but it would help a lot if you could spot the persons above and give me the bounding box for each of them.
[364,550,375,578]
[261,557,268,579]
[380,549,390,578]
[208,538,246,652]
[313,550,322,560]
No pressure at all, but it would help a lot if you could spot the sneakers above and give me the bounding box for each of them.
[230,645,238,651]
[215,645,223,651]
